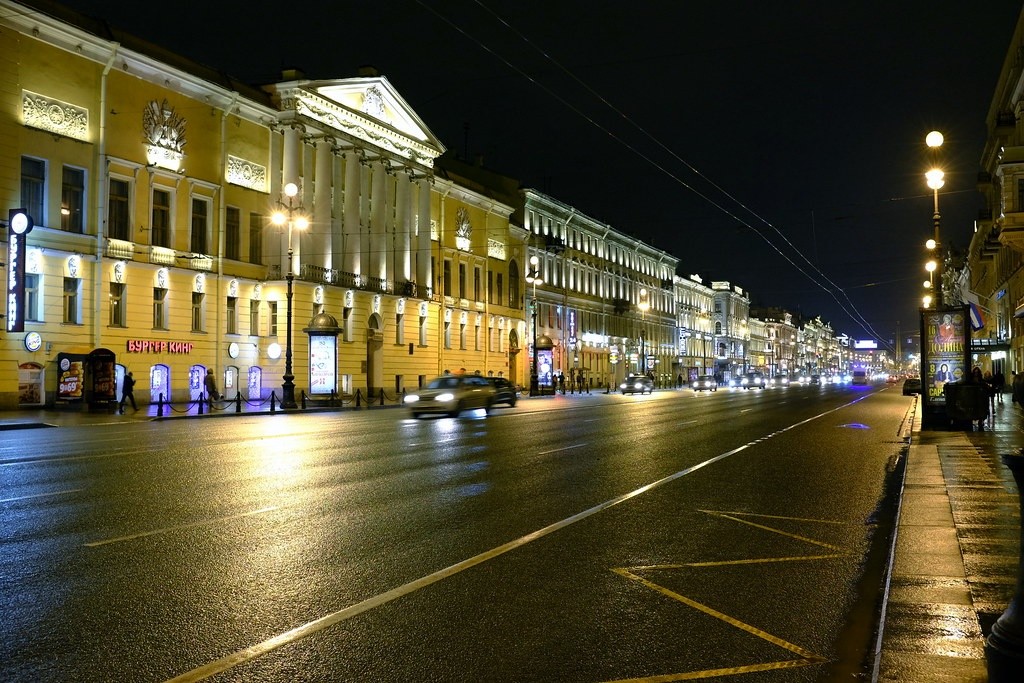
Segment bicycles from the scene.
[515,385,529,395]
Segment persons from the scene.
[970,367,989,432]
[933,364,957,388]
[678,375,682,388]
[953,367,966,383]
[995,369,1005,404]
[983,370,996,414]
[553,375,557,389]
[1012,371,1024,409]
[537,353,549,370]
[558,372,565,391]
[939,314,955,342]
[119,372,140,411]
[204,368,217,410]
[543,371,552,386]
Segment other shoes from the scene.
[134,408,140,411]
[119,407,123,414]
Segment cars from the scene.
[729,377,745,389]
[484,377,517,407]
[742,372,766,390]
[770,375,790,387]
[799,367,919,387]
[620,376,654,395]
[404,376,496,419]
[693,375,718,392]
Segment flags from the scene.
[969,302,987,329]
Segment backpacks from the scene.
[552,376,557,382]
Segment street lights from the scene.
[926,239,936,309]
[527,256,542,397]
[700,308,710,374]
[926,131,945,310]
[790,335,795,377]
[638,289,649,375]
[273,182,309,409]
[770,328,774,378]
[739,319,748,374]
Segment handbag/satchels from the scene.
[212,391,220,401]
